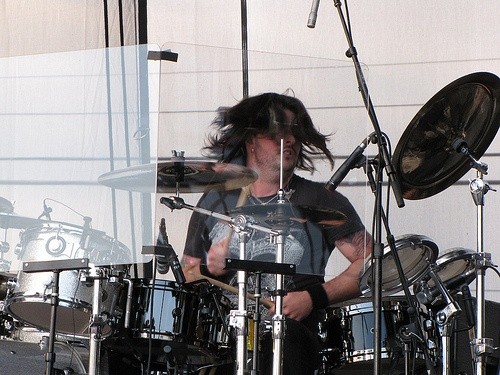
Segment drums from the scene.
[308,295,420,372]
[413,246,492,304]
[8,222,133,339]
[118,277,233,367]
[358,233,440,296]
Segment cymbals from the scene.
[97,158,260,193]
[391,71,500,201]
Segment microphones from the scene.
[44,200,51,221]
[307,0,320,29]
[156,218,170,274]
[325,136,370,193]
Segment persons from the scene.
[180,93,376,375]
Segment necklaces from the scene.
[246,185,288,206]
